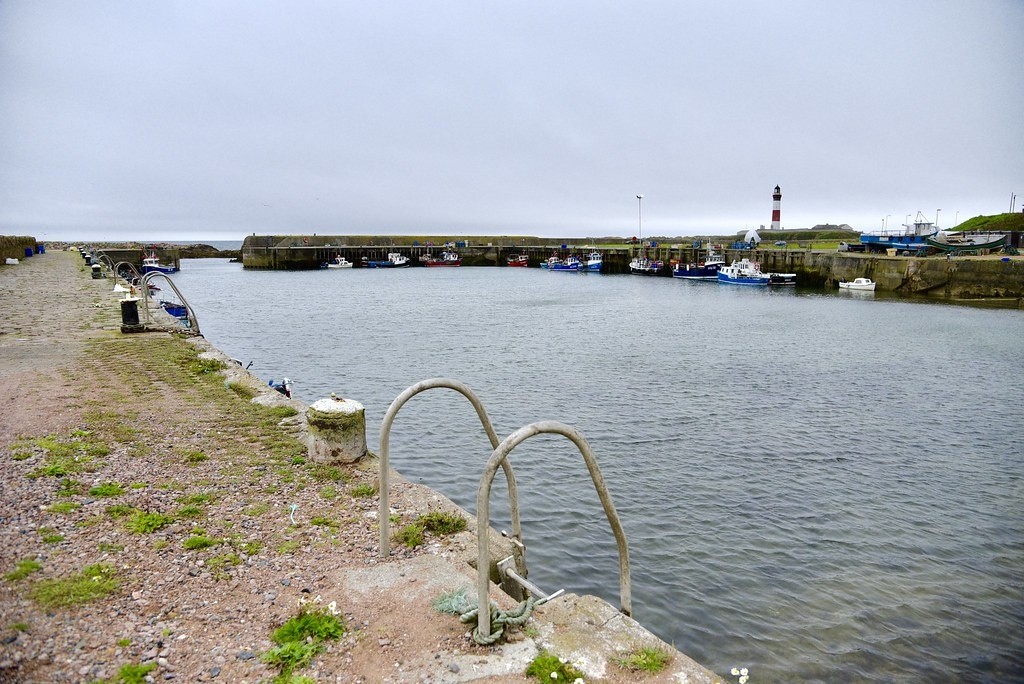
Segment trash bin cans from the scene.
[465,240,468,247]
[562,244,566,248]
[37,245,45,254]
[886,249,897,257]
[25,248,33,257]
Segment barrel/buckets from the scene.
[38,245,44,254]
[26,248,33,256]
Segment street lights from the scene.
[885,214,892,230]
[637,194,645,245]
[955,211,959,225]
[936,209,941,225]
[906,214,911,224]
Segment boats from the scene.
[858,219,942,253]
[143,251,178,275]
[120,270,189,327]
[506,253,529,266]
[672,238,726,280]
[927,230,1008,253]
[539,238,604,272]
[629,253,673,278]
[328,255,353,268]
[839,278,876,291]
[369,252,411,268]
[425,251,464,267]
[767,273,798,285]
[717,258,770,286]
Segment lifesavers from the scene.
[648,263,651,267]
[920,236,925,240]
[910,237,914,241]
[889,235,894,241]
[632,237,637,241]
[898,237,903,241]
[754,265,759,270]
[589,253,592,257]
[303,237,308,243]
